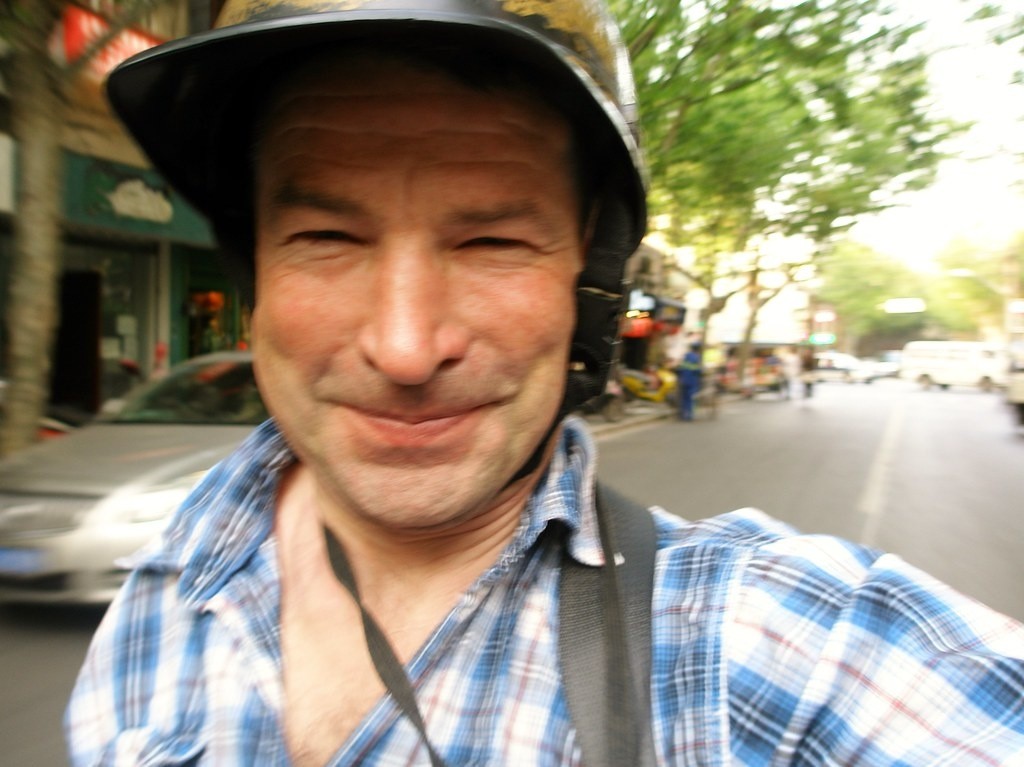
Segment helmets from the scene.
[101,0,649,417]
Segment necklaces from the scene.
[324,478,628,766]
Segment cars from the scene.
[0,346,268,611]
[815,352,899,385]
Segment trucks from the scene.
[901,340,1013,392]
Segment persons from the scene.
[676,342,704,420]
[66,0,1024,766]
